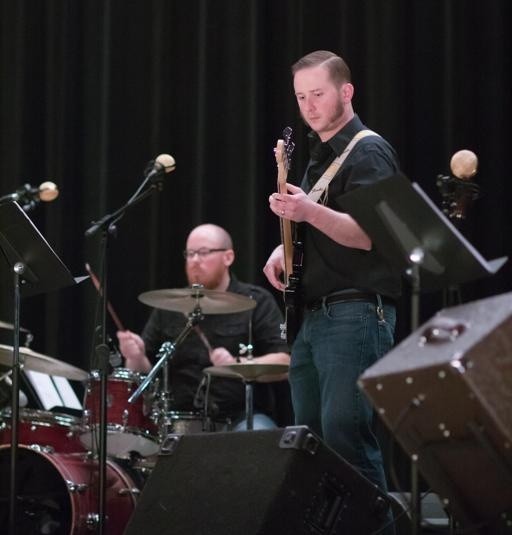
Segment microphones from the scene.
[146,154,177,180]
[450,149,479,218]
[20,181,59,211]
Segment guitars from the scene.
[273,126,306,343]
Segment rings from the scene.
[280,209,285,216]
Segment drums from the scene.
[0,443,137,535]
[134,412,230,473]
[0,404,83,452]
[79,369,164,459]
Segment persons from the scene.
[259,47,409,533]
[115,221,291,439]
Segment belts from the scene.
[304,291,397,312]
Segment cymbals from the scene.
[137,287,257,316]
[204,364,290,381]
[0,321,30,333]
[1,344,89,382]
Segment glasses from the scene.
[183,246,227,260]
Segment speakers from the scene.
[123,426,412,535]
[358,293,511,534]
